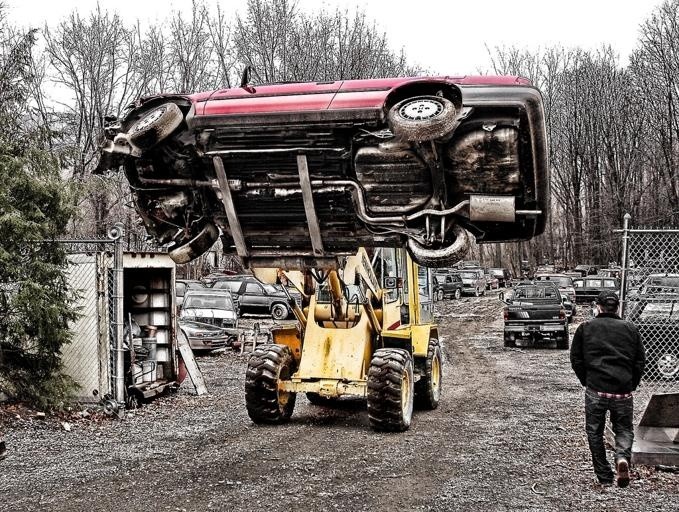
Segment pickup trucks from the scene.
[498,280,570,350]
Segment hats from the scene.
[594,289,620,306]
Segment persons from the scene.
[570,290,645,487]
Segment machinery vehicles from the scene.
[244,243,443,434]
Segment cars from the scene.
[103,66,553,267]
[625,292,679,378]
[176,263,513,321]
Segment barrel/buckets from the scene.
[133,364,143,385]
[144,324,158,338]
[132,337,142,362]
[142,337,158,361]
[142,363,158,383]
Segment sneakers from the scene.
[593,475,612,488]
[616,458,632,489]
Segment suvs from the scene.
[174,288,239,351]
[533,264,679,311]
